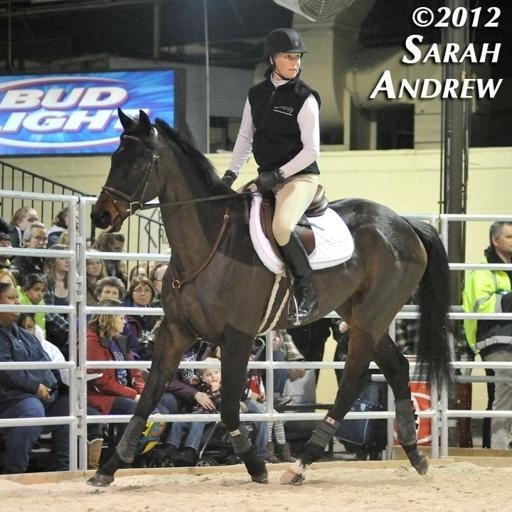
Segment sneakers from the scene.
[127,445,196,467]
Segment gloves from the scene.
[254,168,284,197]
[221,169,237,187]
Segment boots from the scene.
[279,231,320,323]
[86,438,104,469]
[265,442,297,462]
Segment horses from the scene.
[87,106,462,487]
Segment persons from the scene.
[219,25,324,319]
[462,221,512,450]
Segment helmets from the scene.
[263,27,311,58]
